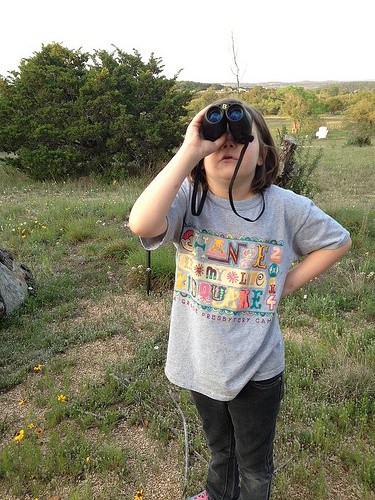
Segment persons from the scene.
[129,99,352,499]
